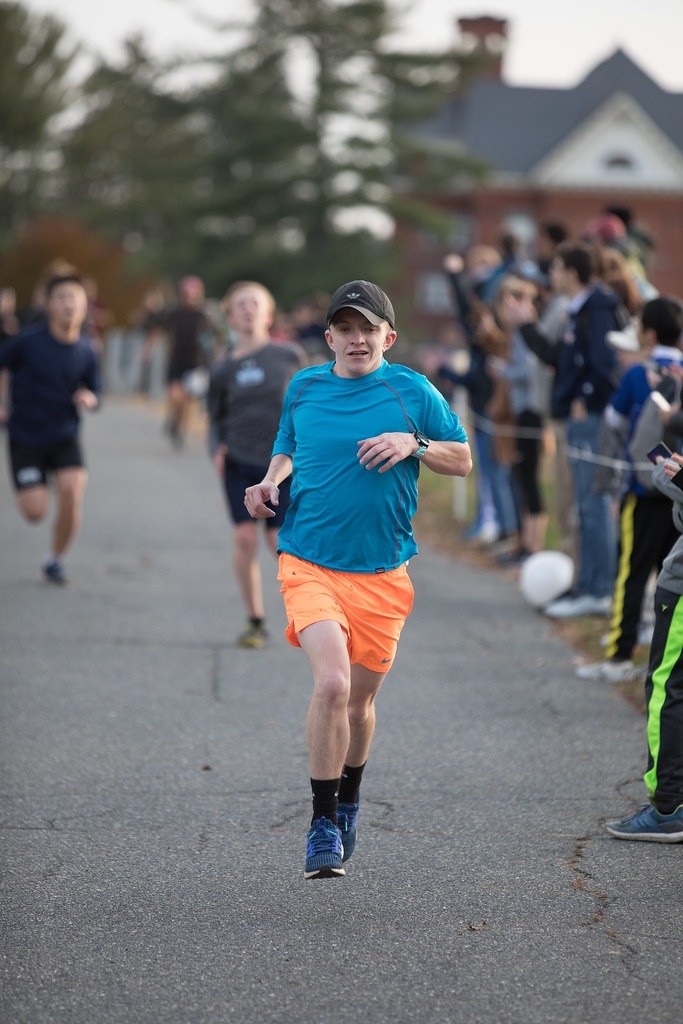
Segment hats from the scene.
[326,280,396,328]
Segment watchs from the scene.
[410,429,429,458]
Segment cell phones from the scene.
[646,441,673,466]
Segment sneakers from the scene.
[304,815,346,879]
[336,792,359,863]
[607,804,683,843]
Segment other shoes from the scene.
[237,617,269,650]
[546,595,613,619]
[577,660,634,684]
[42,564,64,582]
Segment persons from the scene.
[139,202,683,880]
[1,260,106,588]
